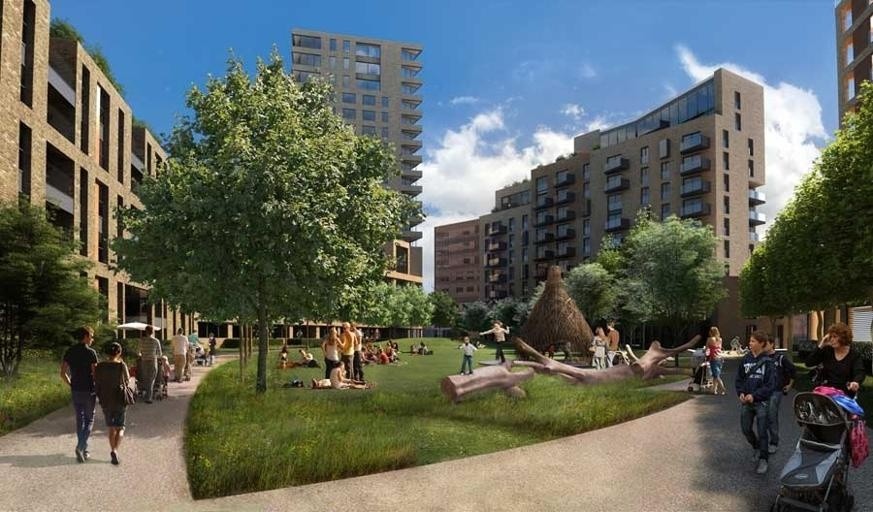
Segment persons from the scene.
[62,325,219,466]
[280,318,872,511]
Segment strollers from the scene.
[195,343,210,368]
[131,356,169,401]
[686,344,726,394]
[175,352,193,381]
[770,384,862,511]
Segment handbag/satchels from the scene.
[116,376,137,406]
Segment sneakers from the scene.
[75,447,90,463]
[768,443,777,454]
[751,448,761,462]
[111,449,120,466]
[756,458,769,475]
